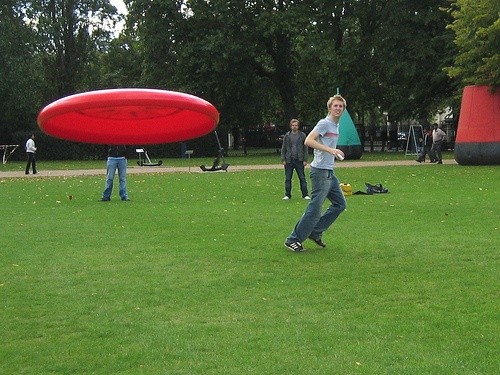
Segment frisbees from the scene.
[37,88,219,146]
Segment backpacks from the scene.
[366,183,388,193]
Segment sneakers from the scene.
[284,240,306,252]
[433,160,438,163]
[283,196,289,200]
[437,161,442,164]
[122,197,130,201]
[304,195,311,200]
[98,197,110,201]
[309,235,326,248]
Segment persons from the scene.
[98,143,130,202]
[24,134,39,174]
[284,95,347,252]
[416,126,433,162]
[281,119,311,199]
[430,123,446,164]
